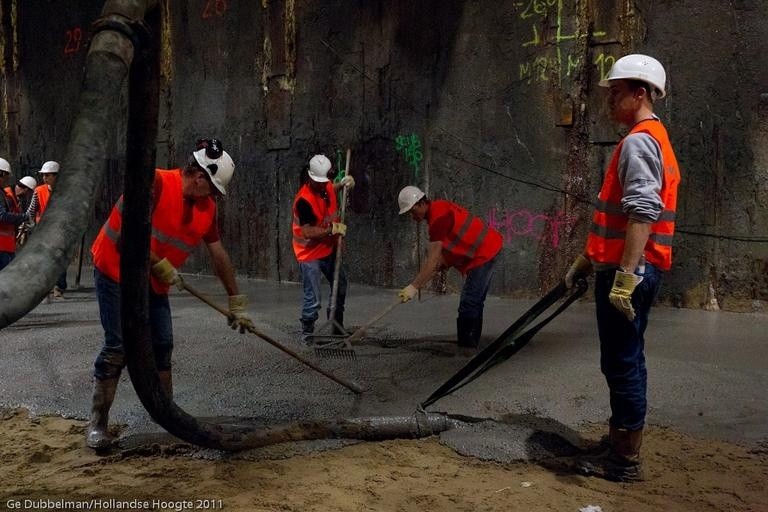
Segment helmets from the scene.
[597,49,671,97]
[37,158,59,175]
[19,173,39,192]
[305,152,332,183]
[0,158,14,175]
[192,144,240,200]
[391,184,426,215]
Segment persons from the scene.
[0,157,28,268]
[395,184,504,348]
[565,52,682,480]
[291,154,355,337]
[87,144,253,448]
[10,176,36,212]
[21,161,67,297]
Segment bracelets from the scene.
[617,265,627,272]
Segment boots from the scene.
[456,316,484,347]
[326,304,347,336]
[602,423,647,483]
[52,285,66,303]
[86,374,119,448]
[301,315,317,348]
[155,371,200,425]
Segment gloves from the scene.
[564,252,595,289]
[609,269,645,322]
[396,282,421,303]
[226,293,256,334]
[148,256,185,292]
[331,220,348,237]
